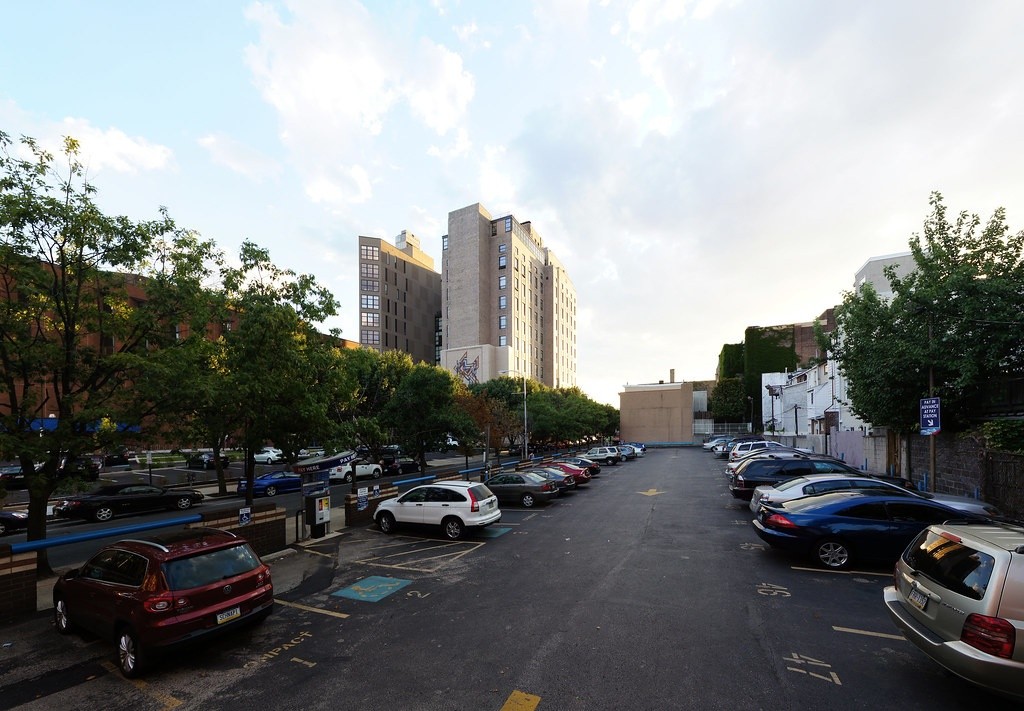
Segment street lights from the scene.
[766,384,781,436]
[748,397,754,423]
[498,369,531,462]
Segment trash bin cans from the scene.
[85,464,99,482]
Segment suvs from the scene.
[577,446,622,466]
[882,519,1022,695]
[376,481,505,540]
[187,450,230,471]
[729,453,920,502]
[48,526,273,681]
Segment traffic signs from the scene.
[921,397,945,437]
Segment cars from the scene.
[624,442,649,452]
[536,461,591,486]
[617,447,635,462]
[51,482,205,521]
[100,446,130,466]
[480,472,559,507]
[0,512,28,534]
[0,466,45,489]
[33,456,100,481]
[251,448,289,465]
[523,465,577,496]
[622,444,644,457]
[749,487,994,571]
[749,474,1001,520]
[331,457,384,484]
[702,433,846,485]
[293,447,328,459]
[556,457,602,477]
[236,470,302,498]
[374,455,423,475]
